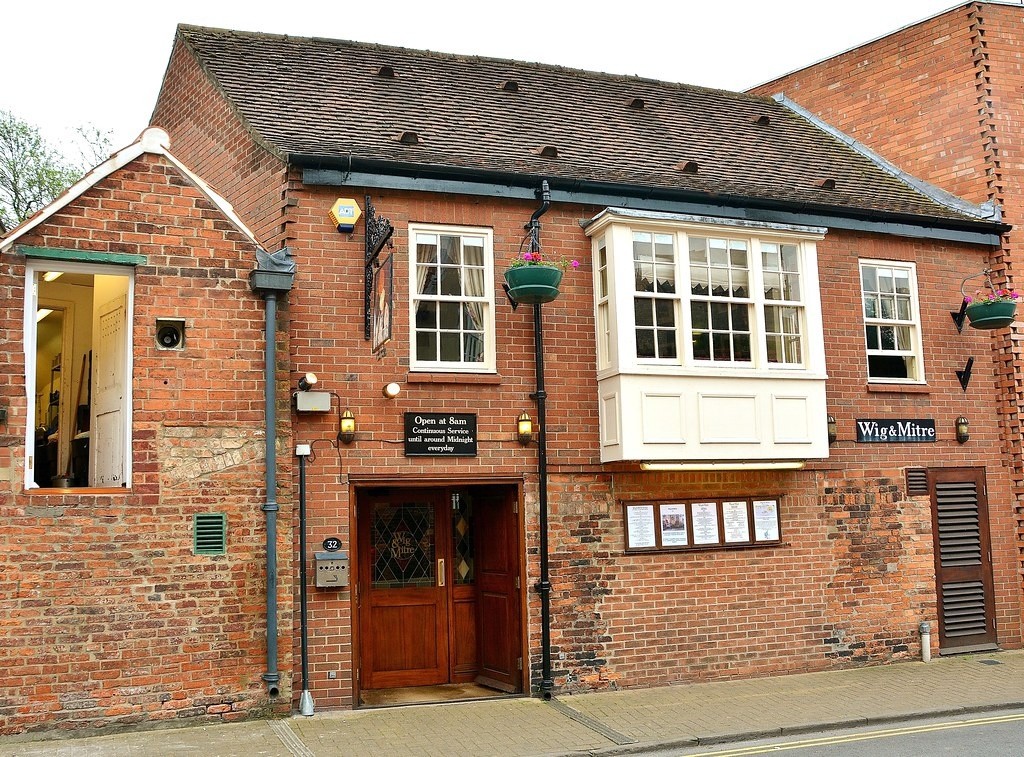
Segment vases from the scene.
[964,299,1017,330]
[503,265,563,304]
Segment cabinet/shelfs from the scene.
[47,363,63,436]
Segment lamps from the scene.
[516,409,533,447]
[827,413,837,446]
[337,408,356,446]
[298,373,318,392]
[955,414,969,445]
[382,381,400,400]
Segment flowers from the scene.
[509,253,580,271]
[963,288,1019,306]
